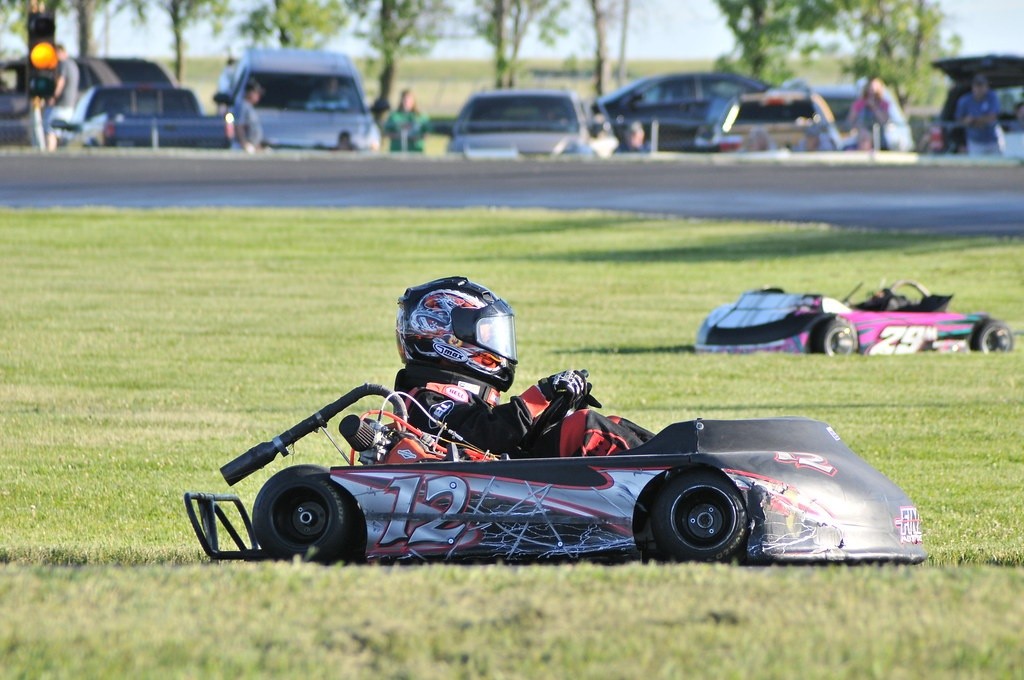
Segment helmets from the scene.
[394,274,519,393]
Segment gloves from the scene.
[540,369,587,399]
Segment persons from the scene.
[26,44,80,147]
[395,276,655,458]
[790,125,832,150]
[846,78,889,149]
[338,132,355,150]
[845,129,875,149]
[615,122,650,152]
[214,59,235,113]
[231,79,262,150]
[956,76,1001,155]
[736,125,775,153]
[1009,103,1024,130]
[384,91,431,151]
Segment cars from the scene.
[422,90,612,162]
[719,91,839,151]
[597,72,776,156]
[921,55,1024,160]
[1,56,175,147]
[40,85,227,151]
[214,47,382,158]
[783,83,908,153]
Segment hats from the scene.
[973,72,985,85]
